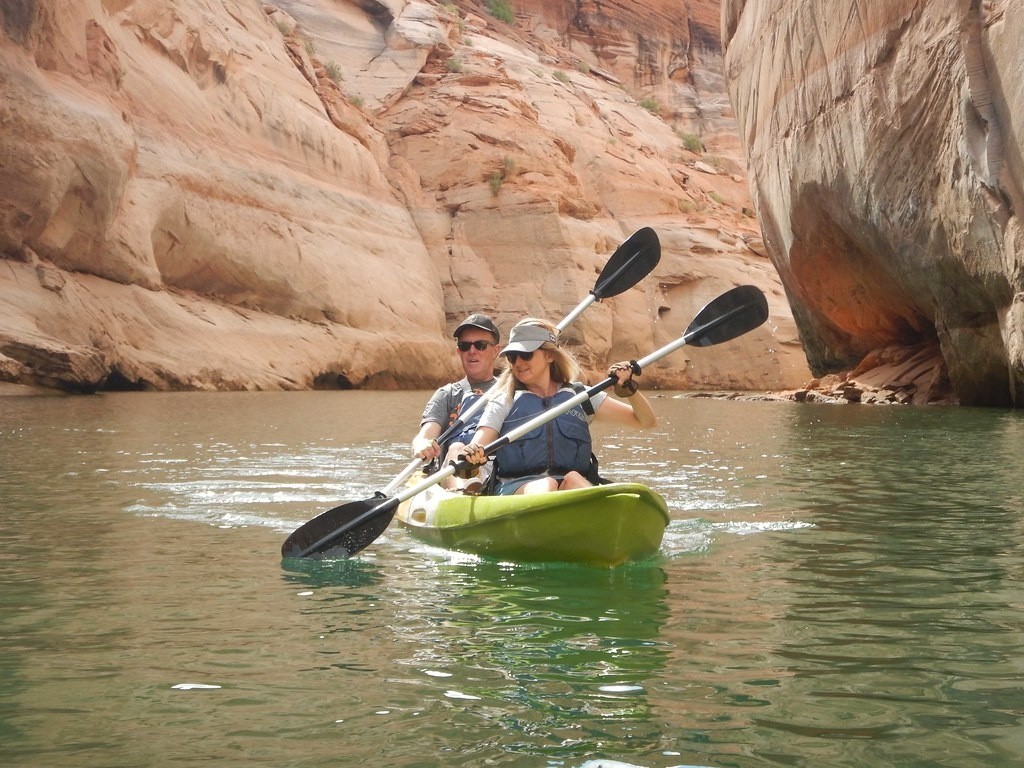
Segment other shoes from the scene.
[463,477,482,496]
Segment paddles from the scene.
[281,284,770,562]
[368,224,663,501]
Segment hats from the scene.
[453,315,500,345]
[498,325,556,358]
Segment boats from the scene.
[392,458,671,571]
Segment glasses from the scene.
[457,340,495,353]
[505,349,547,363]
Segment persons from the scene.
[458,318,657,495]
[412,314,502,496]
